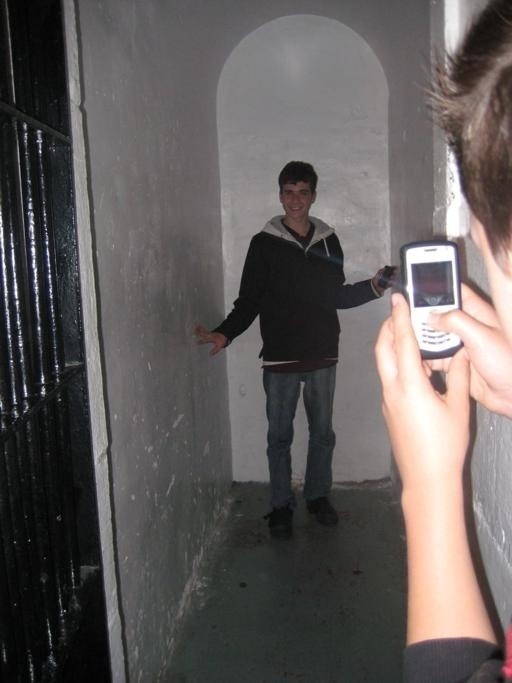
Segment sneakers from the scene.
[307,497,338,526]
[271,508,292,539]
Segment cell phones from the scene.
[378,265,394,288]
[400,241,464,359]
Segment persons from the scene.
[374,0,512,683]
[192,160,398,542]
